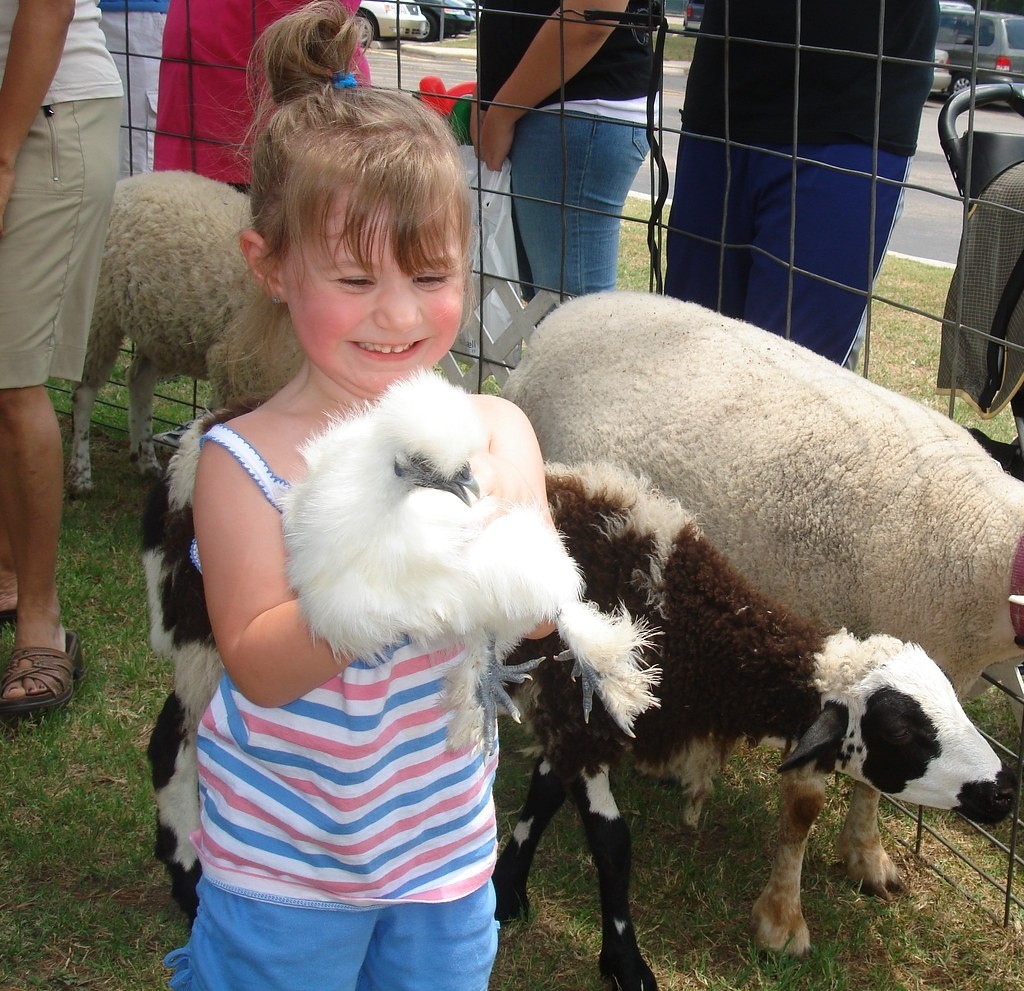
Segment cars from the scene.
[927,48,953,95]
[682,0,705,37]
[934,9,1024,101]
[938,1,975,12]
[353,0,483,47]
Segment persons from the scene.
[168,8,559,991]
[99,0,169,179]
[662,0,940,372]
[470,0,668,304]
[153,0,369,184]
[0,0,123,708]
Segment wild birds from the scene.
[277,368,664,768]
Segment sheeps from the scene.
[138,401,1019,991]
[66,169,305,503]
[502,288,1024,958]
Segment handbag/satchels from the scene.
[451,143,523,367]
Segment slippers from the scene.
[0,631,84,714]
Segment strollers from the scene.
[936,79,1024,483]
